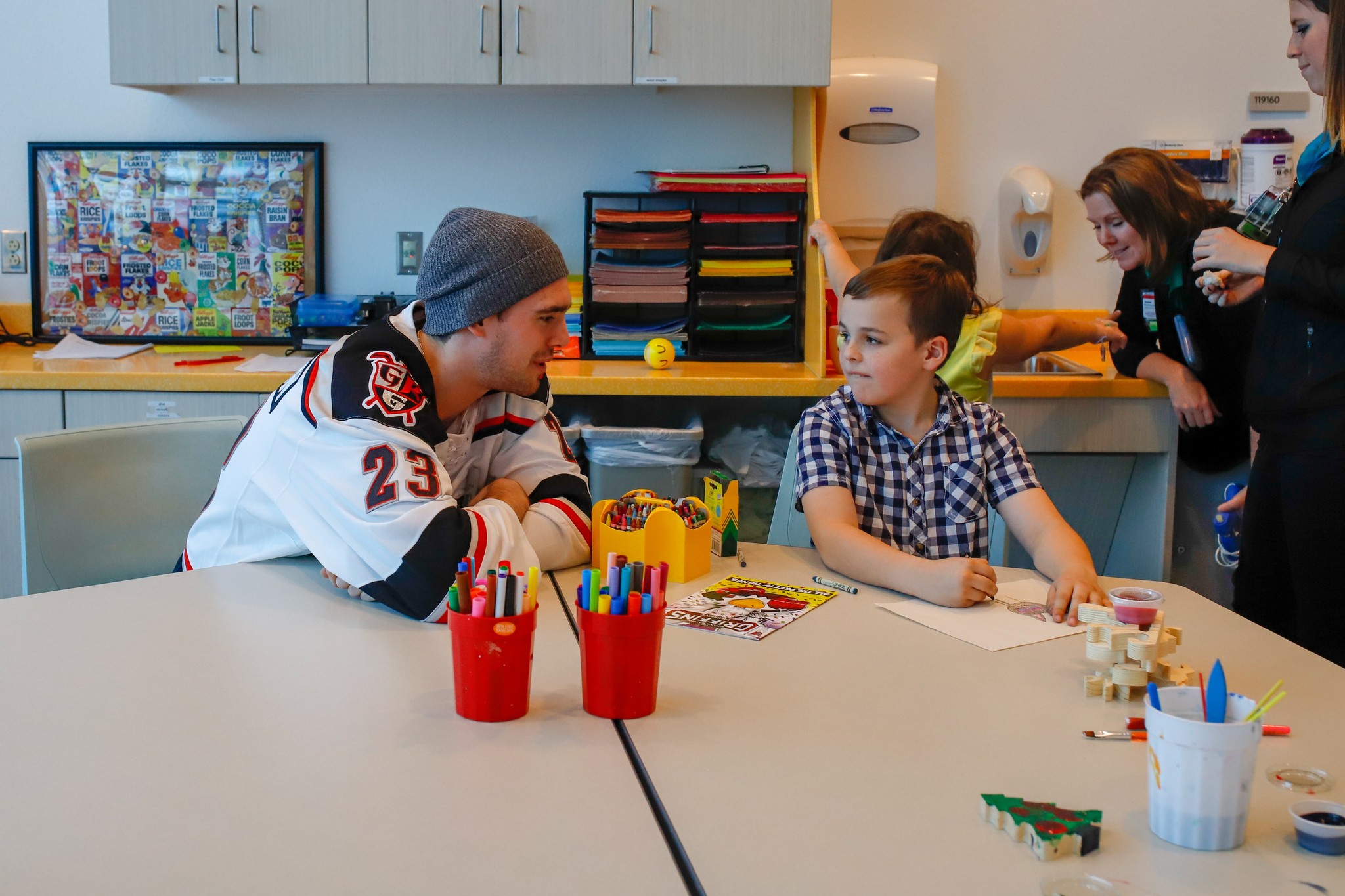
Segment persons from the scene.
[173,206,591,624]
[1081,149,1274,511]
[808,212,1126,405]
[796,254,1112,626]
[1191,0,1344,665]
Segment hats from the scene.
[414,206,571,338]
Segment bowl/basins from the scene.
[1108,587,1166,625]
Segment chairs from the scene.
[767,419,1011,568]
[13,412,250,598]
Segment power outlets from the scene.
[1,230,27,274]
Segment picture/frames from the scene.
[27,142,325,346]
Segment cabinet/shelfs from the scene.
[366,0,633,85]
[0,390,276,601]
[580,190,809,362]
[107,0,367,85]
[632,0,832,86]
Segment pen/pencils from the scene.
[1126,717,1290,736]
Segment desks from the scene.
[551,539,1345,896]
[0,553,692,896]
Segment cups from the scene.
[1143,686,1263,851]
[573,597,668,720]
[446,599,539,723]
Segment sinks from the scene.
[987,347,1108,380]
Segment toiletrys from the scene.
[996,165,1056,279]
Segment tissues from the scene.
[812,55,943,258]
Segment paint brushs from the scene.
[1083,731,1147,743]
